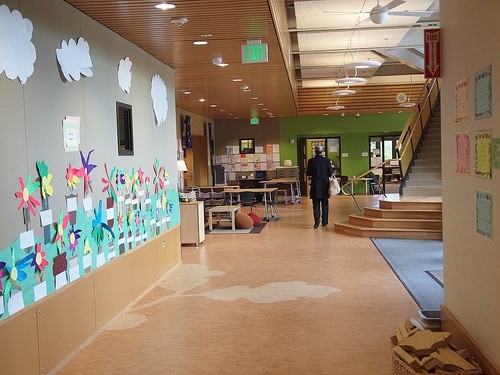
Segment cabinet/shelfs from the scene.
[276,165,299,196]
[186,185,241,223]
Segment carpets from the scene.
[204,222,267,234]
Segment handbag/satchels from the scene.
[328,173,340,196]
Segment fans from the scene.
[322,0,434,26]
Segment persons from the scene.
[306,146,332,229]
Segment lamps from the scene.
[332,63,356,96]
[177,159,188,192]
[398,74,416,108]
[341,20,381,70]
[325,85,346,110]
[334,43,366,84]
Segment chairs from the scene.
[368,174,381,195]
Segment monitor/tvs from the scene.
[256,171,266,178]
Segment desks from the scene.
[222,189,278,222]
[348,178,373,196]
[259,179,301,205]
[209,205,241,232]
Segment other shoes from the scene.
[314,219,320,228]
